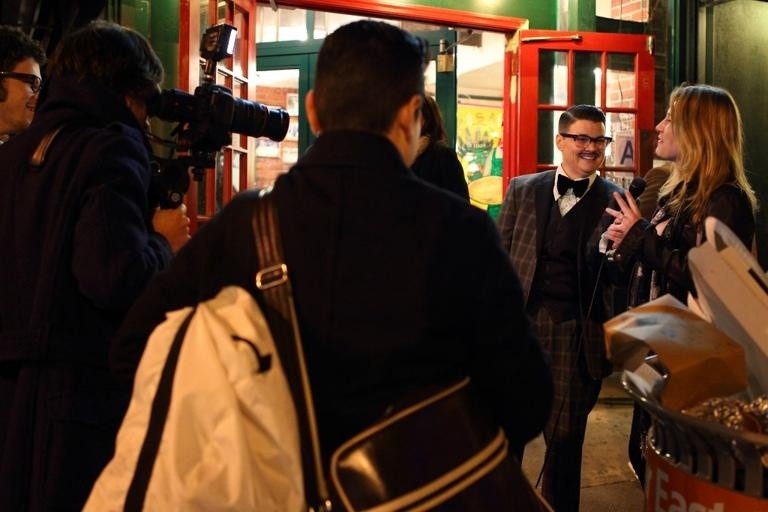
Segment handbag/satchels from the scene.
[308,375,556,512]
[80,188,306,512]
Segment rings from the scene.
[620,215,626,220]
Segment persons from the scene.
[1,19,196,511]
[93,18,555,512]
[497,105,633,512]
[408,95,471,206]
[595,85,759,486]
[0,23,47,143]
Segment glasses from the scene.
[560,132,612,150]
[0,72,41,93]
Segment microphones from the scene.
[606,176,647,253]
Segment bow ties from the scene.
[557,174,589,198]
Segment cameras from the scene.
[154,23,290,172]
